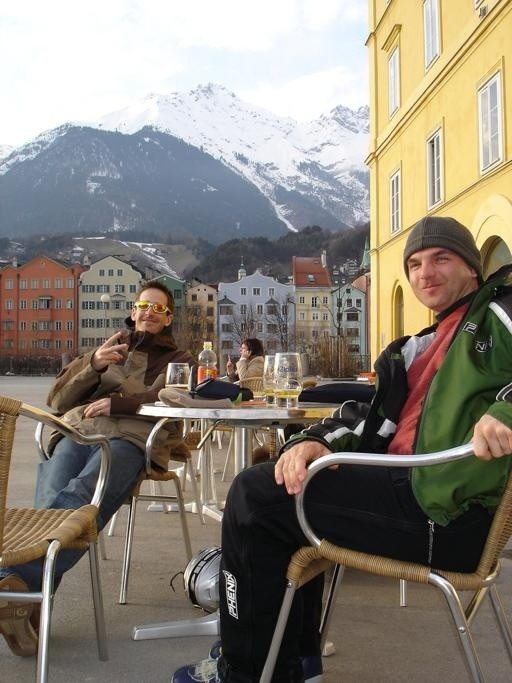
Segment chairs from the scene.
[261,443,510,683]
[1,396,114,683]
[35,410,195,607]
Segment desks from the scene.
[134,395,356,647]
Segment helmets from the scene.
[182,545,223,615]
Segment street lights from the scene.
[337,334,343,377]
[328,334,335,376]
[100,293,112,340]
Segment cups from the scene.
[262,351,303,410]
[165,361,191,392]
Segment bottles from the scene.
[197,340,218,386]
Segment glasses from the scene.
[132,300,173,316]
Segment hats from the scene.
[403,215,485,289]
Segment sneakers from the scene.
[0,573,42,658]
[169,640,325,683]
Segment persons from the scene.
[227,337,265,382]
[0,278,196,658]
[173,216,512,683]
